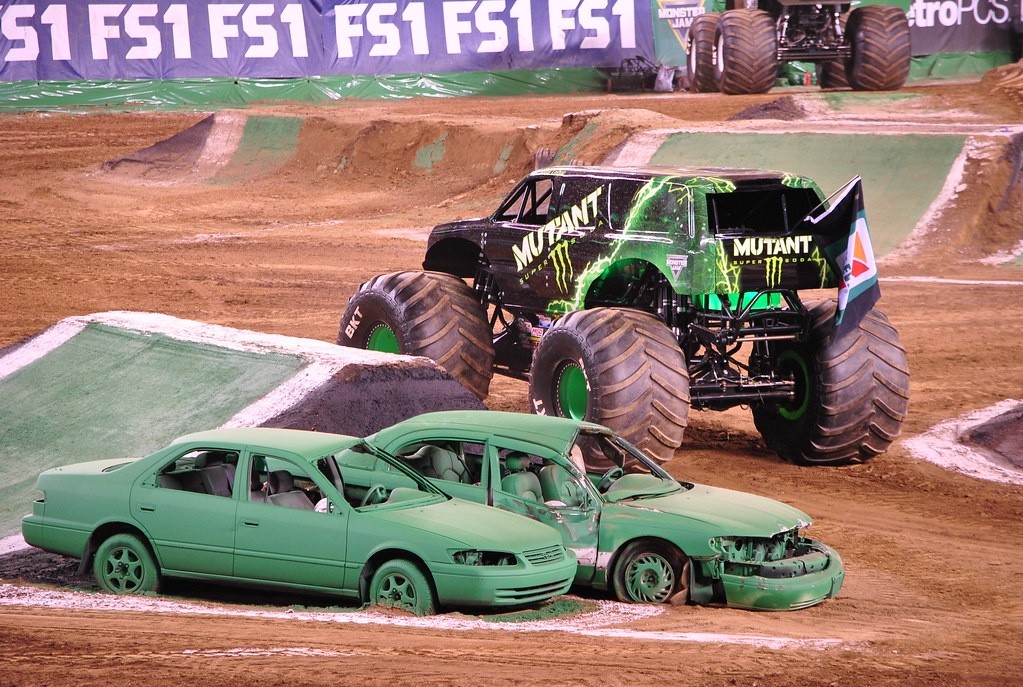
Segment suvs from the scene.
[335,162,910,470]
[686,0,911,94]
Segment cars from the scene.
[21,426,578,614]
[359,410,846,614]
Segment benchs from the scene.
[159,453,267,504]
[399,445,472,486]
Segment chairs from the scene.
[539,458,583,506]
[501,452,545,504]
[266,470,315,510]
